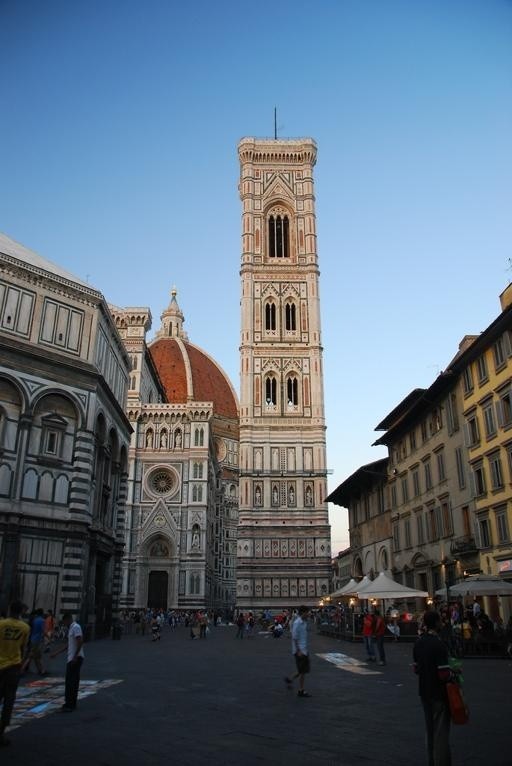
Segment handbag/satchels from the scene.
[447,682,470,725]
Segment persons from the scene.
[1,601,57,746]
[412,610,455,766]
[285,607,313,697]
[371,609,388,665]
[119,606,337,642]
[61,613,86,715]
[361,606,375,666]
[331,599,511,654]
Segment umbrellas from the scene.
[434,573,512,597]
[330,572,429,618]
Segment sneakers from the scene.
[362,657,387,666]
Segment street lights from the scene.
[442,559,458,633]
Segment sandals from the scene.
[284,677,293,689]
[296,689,311,697]
